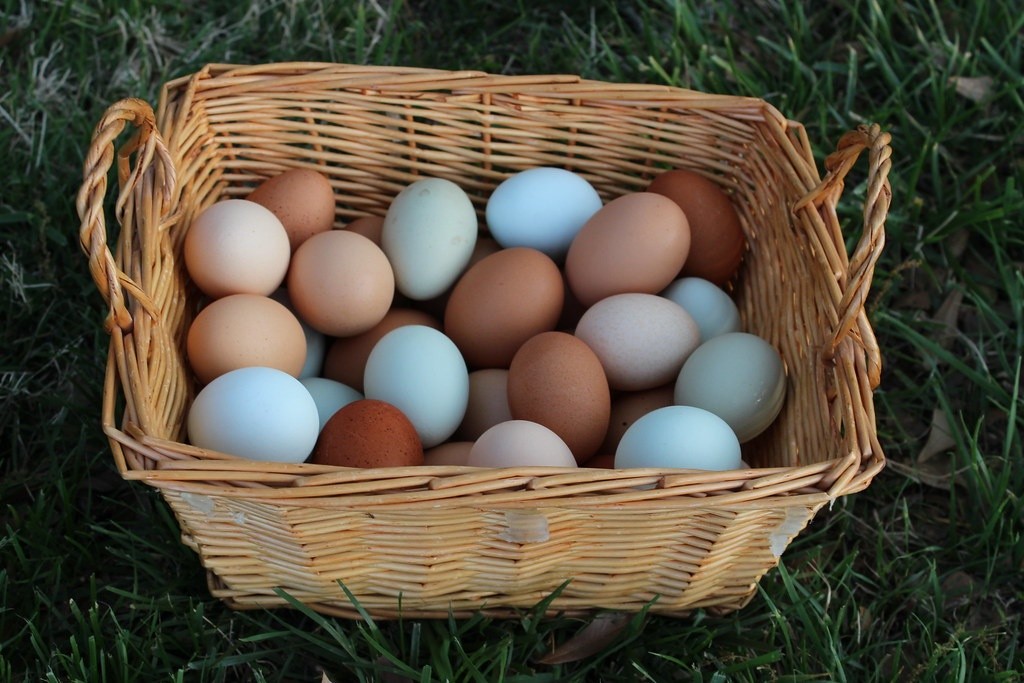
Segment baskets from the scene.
[77,63,892,620]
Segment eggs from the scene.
[183,167,787,471]
